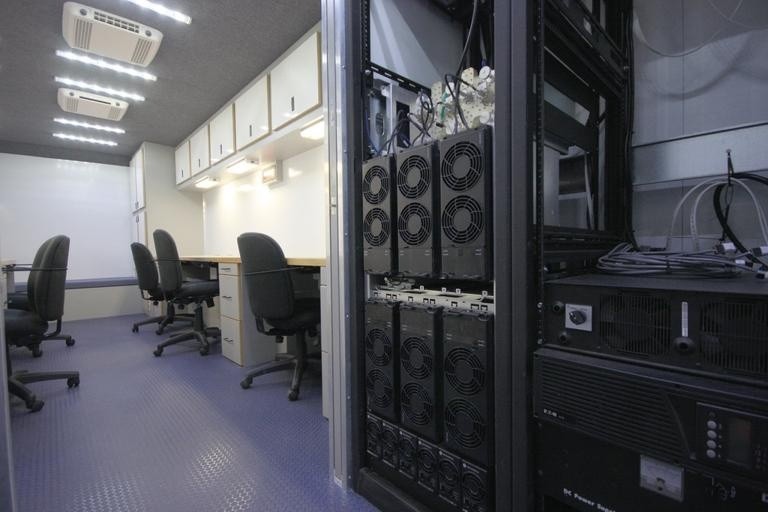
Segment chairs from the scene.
[0,235,81,412]
[128,227,320,402]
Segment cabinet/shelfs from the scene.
[172,19,327,193]
[128,141,206,256]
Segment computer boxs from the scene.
[437,126,491,283]
[543,272,768,387]
[361,298,402,426]
[360,152,396,280]
[396,303,441,445]
[439,307,495,475]
[395,142,438,283]
